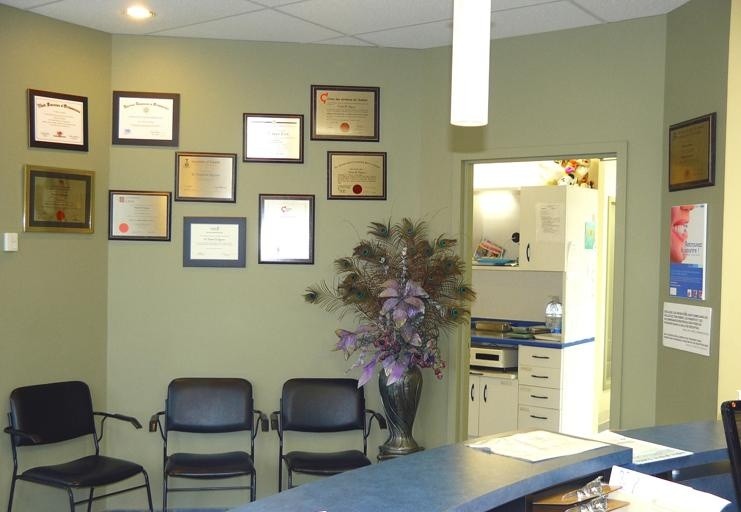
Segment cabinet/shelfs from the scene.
[519,185,565,271]
[467,375,516,438]
[516,345,559,432]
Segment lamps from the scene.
[450,0,492,128]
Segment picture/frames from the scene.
[107,190,170,241]
[258,194,315,264]
[112,91,179,148]
[23,163,95,234]
[665,112,716,192]
[243,112,304,164]
[183,216,247,268]
[174,152,237,204]
[28,88,89,153]
[327,151,387,199]
[309,84,379,142]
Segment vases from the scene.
[376,364,423,463]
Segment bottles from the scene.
[546,297,561,331]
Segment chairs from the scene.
[4,380,154,512]
[149,378,269,512]
[270,377,386,492]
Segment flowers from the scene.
[302,214,478,390]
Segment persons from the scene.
[671,206,698,263]
